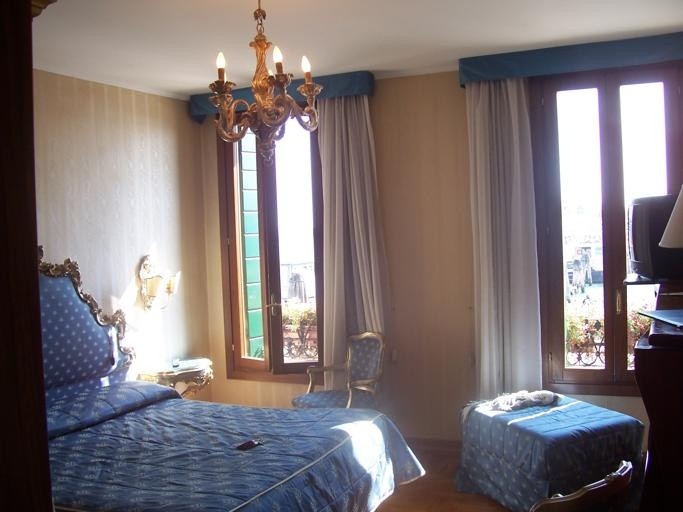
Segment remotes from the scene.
[238,439,261,450]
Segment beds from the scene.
[38,244,414,512]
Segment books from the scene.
[638,309,683,327]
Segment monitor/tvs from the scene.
[627,194,683,281]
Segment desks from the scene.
[136,357,214,400]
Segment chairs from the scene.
[291,332,386,408]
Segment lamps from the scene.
[657,181,683,249]
[136,254,181,312]
[209,0,325,163]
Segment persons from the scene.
[581,249,592,286]
[573,250,584,293]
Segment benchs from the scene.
[453,391,646,510]
